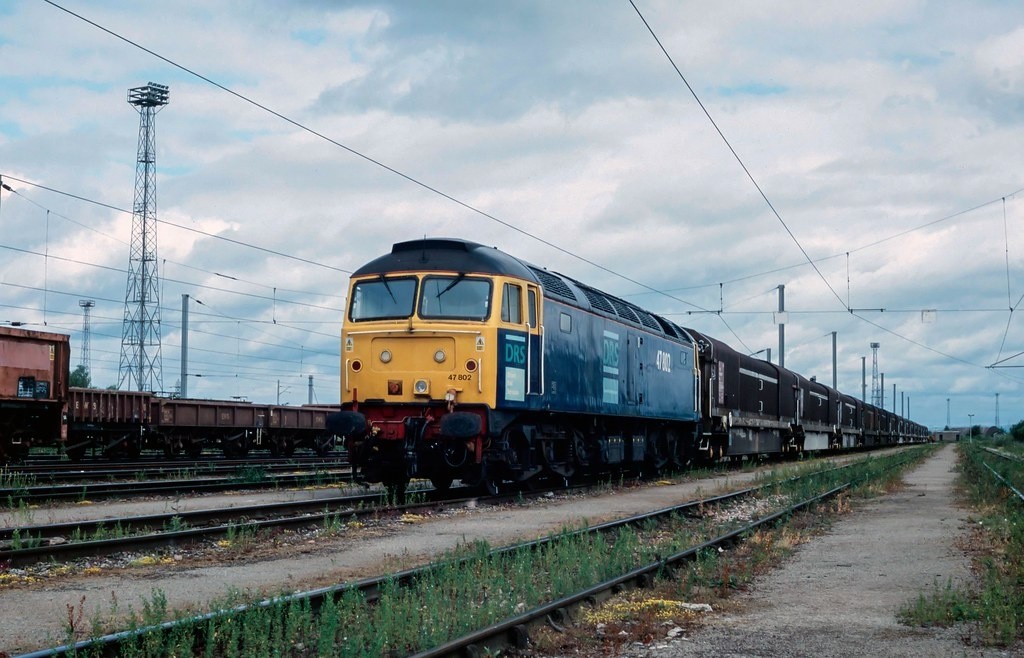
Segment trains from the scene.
[327,236,933,498]
[0,325,344,464]
